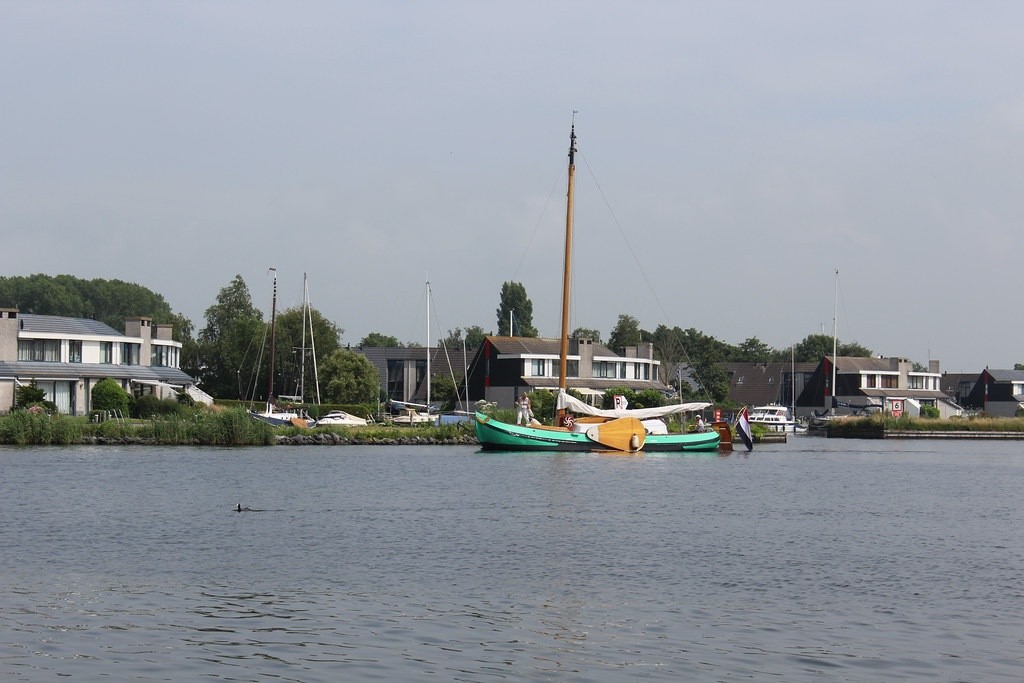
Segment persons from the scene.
[689,414,704,433]
[515,391,531,426]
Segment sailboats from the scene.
[810,264,882,427]
[473,110,721,452]
[237,267,322,429]
[383,271,475,423]
[749,340,807,433]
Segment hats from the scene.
[696,415,701,418]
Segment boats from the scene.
[317,409,369,428]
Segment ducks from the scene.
[236,503,251,512]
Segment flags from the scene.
[736,409,753,451]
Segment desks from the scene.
[689,424,712,434]
